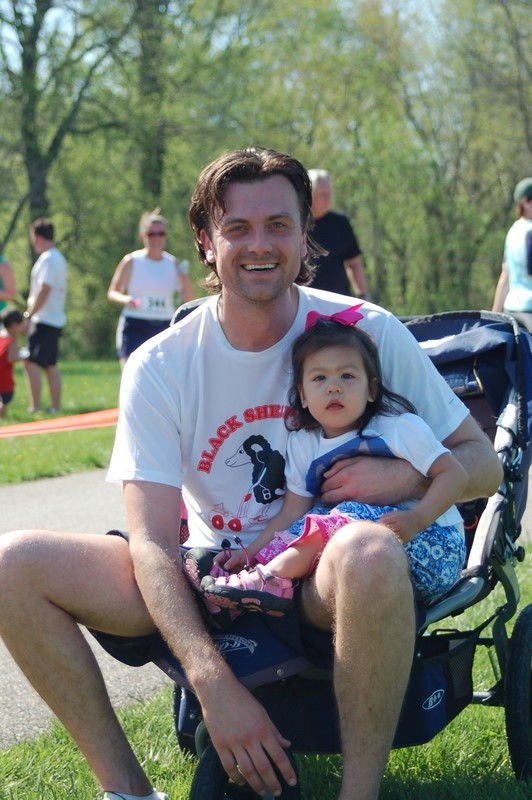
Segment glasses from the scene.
[148,230,166,237]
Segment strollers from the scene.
[173,310,532,800]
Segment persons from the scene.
[305,169,371,304]
[107,208,196,374]
[183,317,467,628]
[0,218,68,415]
[0,147,504,800]
[492,177,532,333]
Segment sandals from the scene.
[183,547,242,628]
[200,536,295,619]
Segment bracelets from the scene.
[23,312,31,318]
